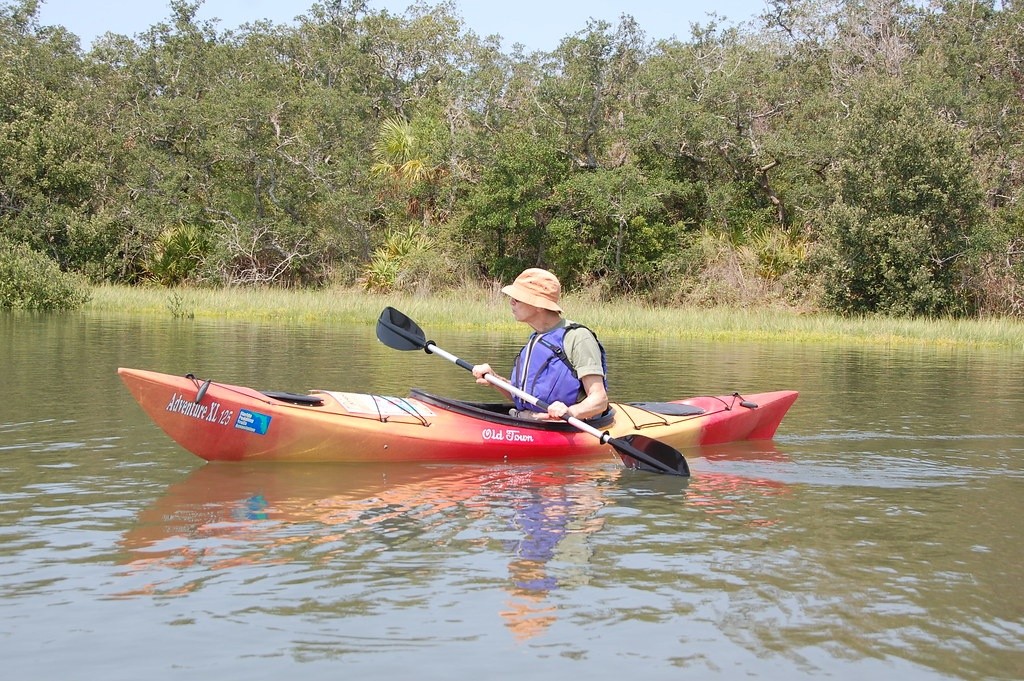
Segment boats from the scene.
[118,365,801,464]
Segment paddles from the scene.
[375,306,691,476]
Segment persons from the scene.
[473,267,609,424]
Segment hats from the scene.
[501,268,564,315]
